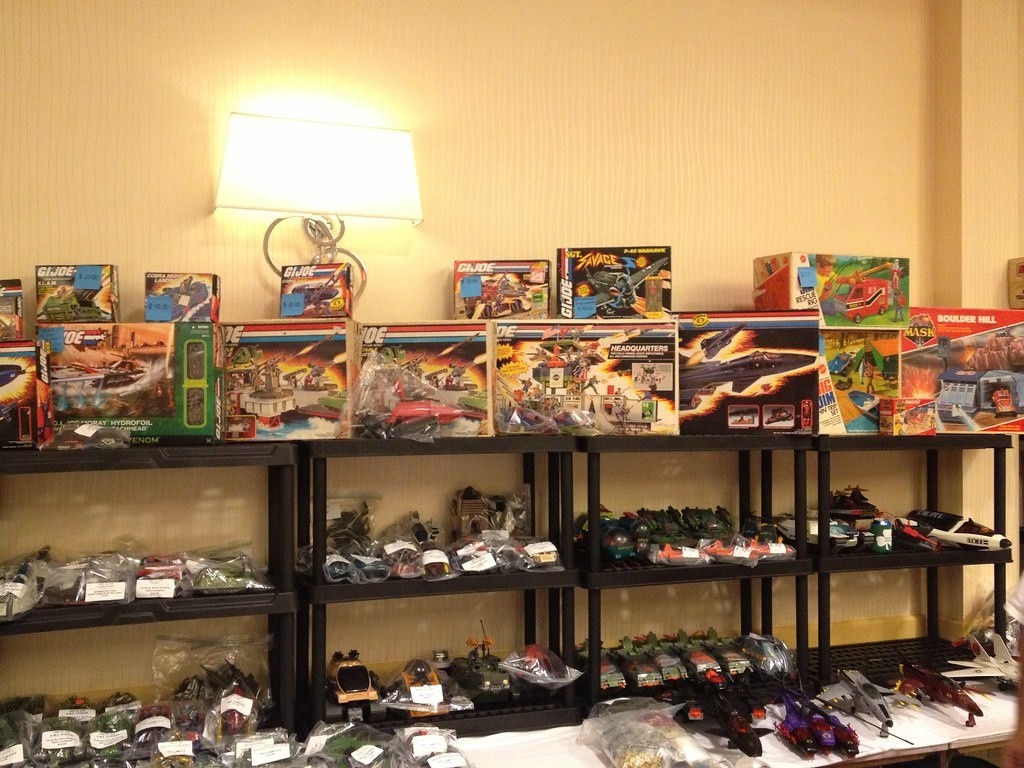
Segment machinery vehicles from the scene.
[326,615,799,727]
[0,485,560,623]
[573,485,1011,567]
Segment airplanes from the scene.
[656,634,1024,760]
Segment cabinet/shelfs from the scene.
[760,434,1014,700]
[561,438,818,721]
[299,436,580,746]
[0,441,298,768]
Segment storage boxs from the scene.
[362,320,498,437]
[279,262,354,318]
[878,398,937,438]
[144,273,222,324]
[35,323,223,450]
[0,340,36,451]
[0,279,23,342]
[901,307,1024,433]
[221,319,363,443]
[557,246,672,319]
[454,260,551,321]
[497,319,681,437]
[672,310,819,437]
[818,330,903,436]
[35,264,120,324]
[1008,257,1024,309]
[753,252,910,328]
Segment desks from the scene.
[748,676,1020,768]
